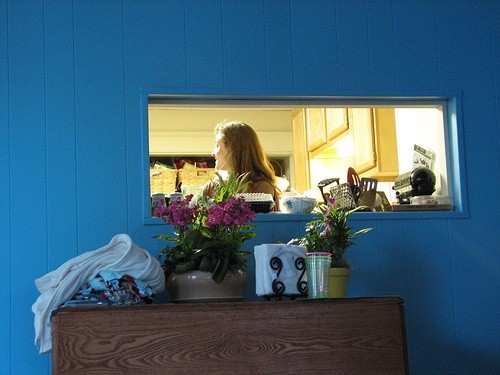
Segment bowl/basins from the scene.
[286,196,316,213]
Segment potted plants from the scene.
[286,198,374,298]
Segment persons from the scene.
[189,120,281,212]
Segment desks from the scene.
[50,296,410,375]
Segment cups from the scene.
[305,252,331,298]
[169,192,183,203]
[152,193,166,209]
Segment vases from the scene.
[165,269,247,302]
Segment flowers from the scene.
[148,172,256,284]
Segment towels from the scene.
[30,232,165,354]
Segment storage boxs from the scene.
[150,161,177,195]
[178,159,216,194]
[254,243,308,301]
